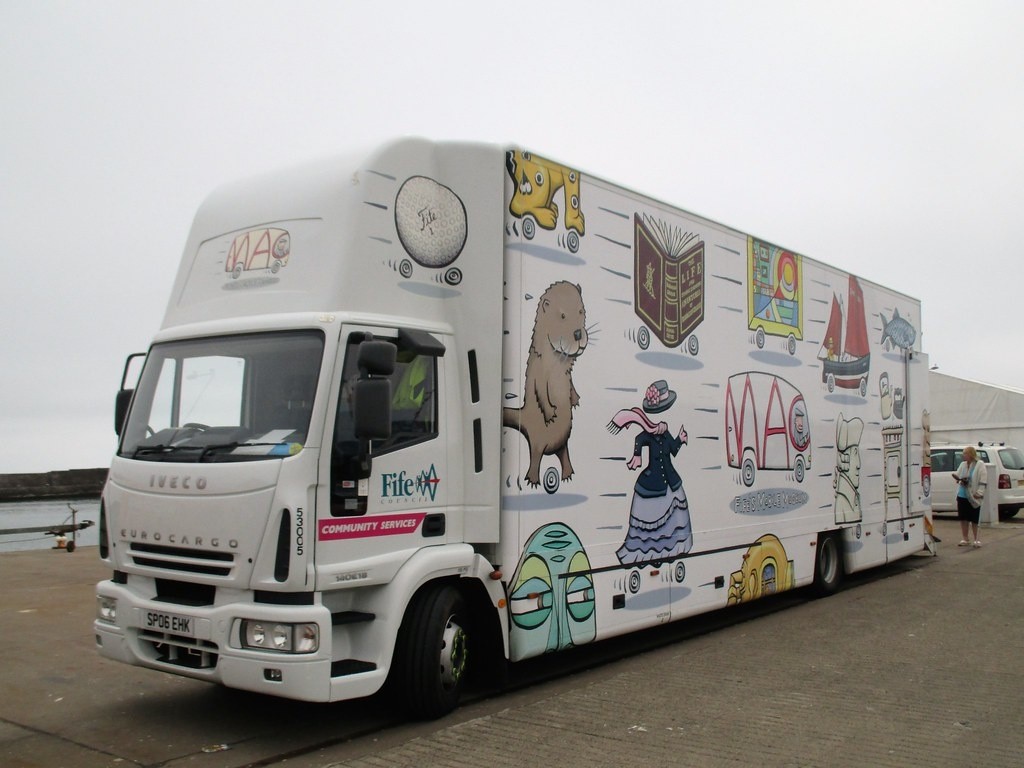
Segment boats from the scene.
[0,520,90,534]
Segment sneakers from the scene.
[973,541,982,548]
[958,541,969,546]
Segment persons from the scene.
[954,447,987,548]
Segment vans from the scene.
[929,442,1024,524]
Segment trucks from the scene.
[90,136,941,719]
